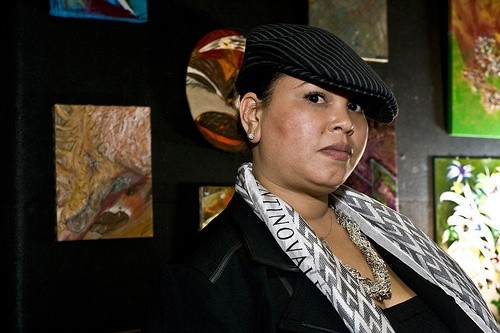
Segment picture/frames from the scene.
[430,155,500,328]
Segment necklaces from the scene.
[315,209,331,242]
[308,204,391,302]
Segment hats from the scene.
[235,23,399,125]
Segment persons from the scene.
[180,22,500,332]
